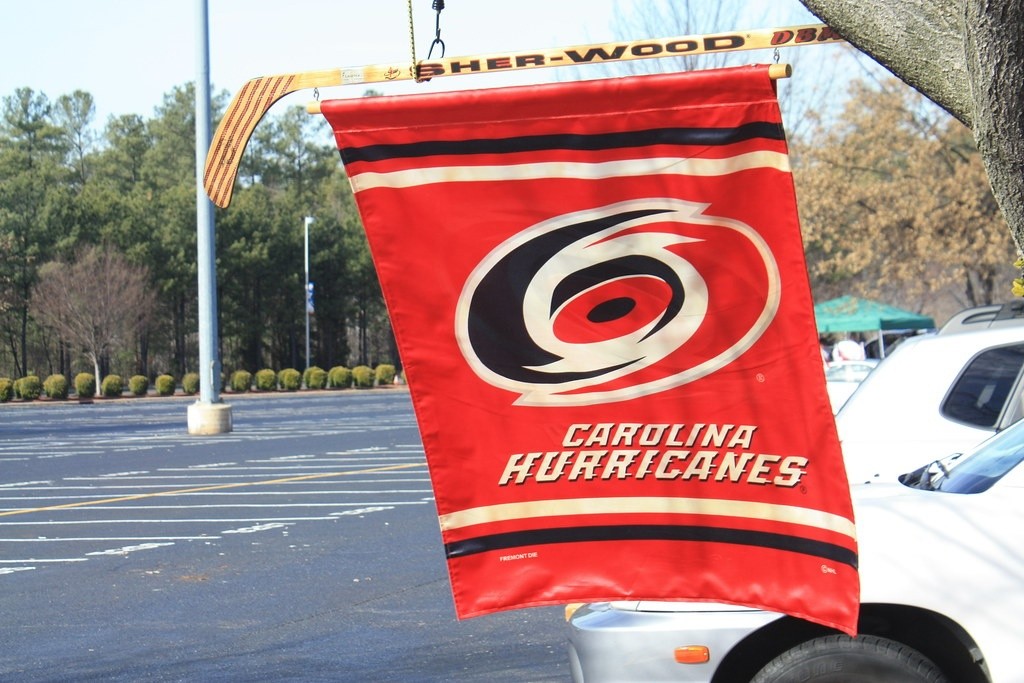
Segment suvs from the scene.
[835,300,1024,474]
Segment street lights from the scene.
[304,216,315,368]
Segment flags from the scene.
[320,64,861,637]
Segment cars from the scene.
[565,421,1024,683]
[824,361,881,413]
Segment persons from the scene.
[819,332,895,363]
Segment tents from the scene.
[813,295,935,359]
